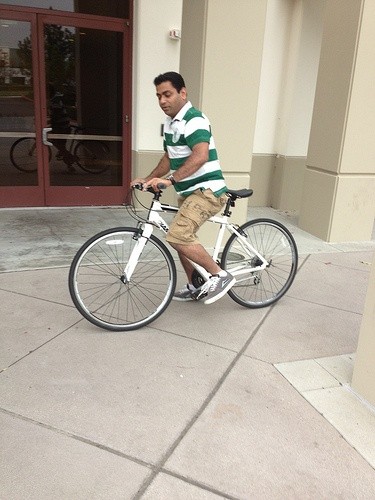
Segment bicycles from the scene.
[9,117,113,175]
[68,183,299,331]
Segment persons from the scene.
[45,83,71,156]
[129,71,238,304]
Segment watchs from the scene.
[166,174,176,185]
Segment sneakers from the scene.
[202,270,236,305]
[171,285,208,301]
